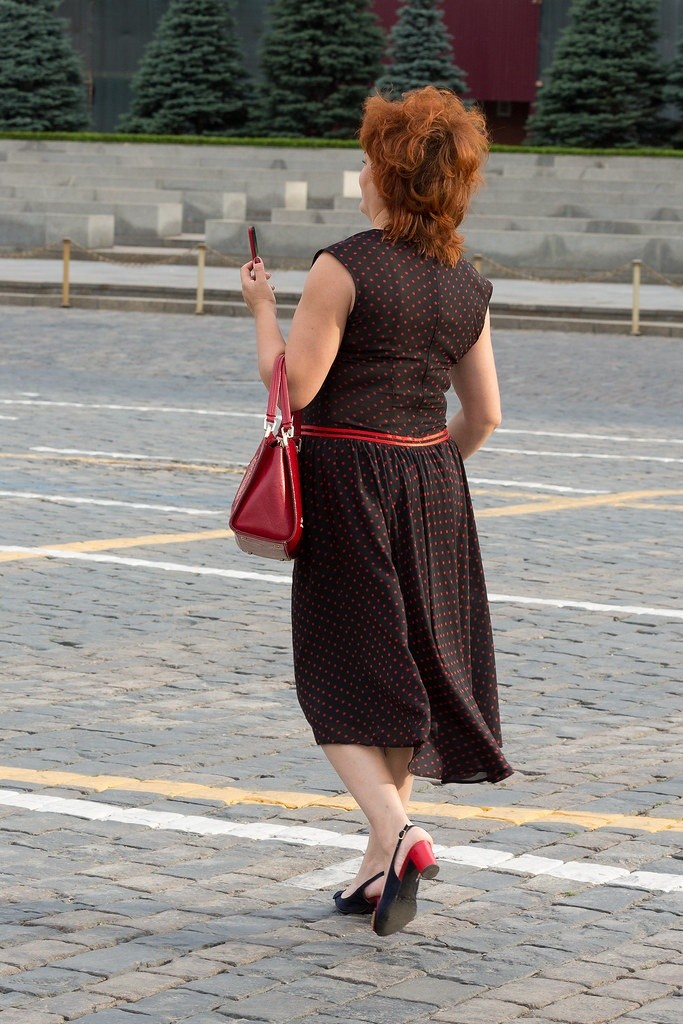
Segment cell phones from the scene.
[246,225,261,264]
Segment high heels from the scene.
[332,869,386,914]
[370,822,440,936]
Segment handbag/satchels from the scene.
[229,353,302,557]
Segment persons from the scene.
[226,87,514,936]
